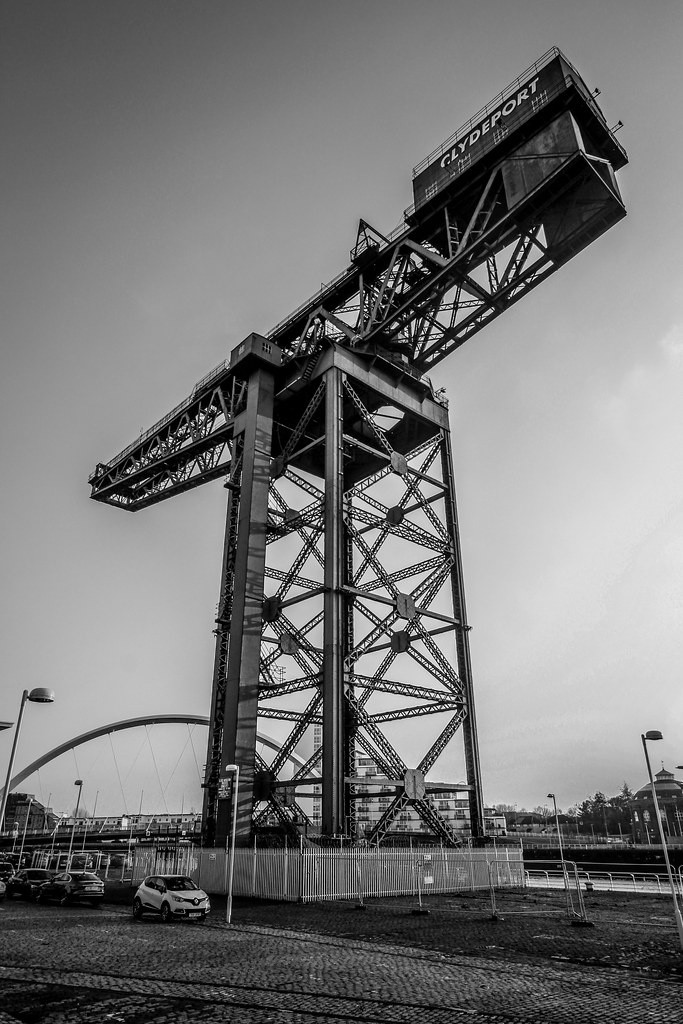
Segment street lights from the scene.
[224,764,239,925]
[8,779,188,882]
[639,729,683,950]
[546,792,570,891]
[0,685,54,841]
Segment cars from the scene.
[0,861,15,888]
[131,874,211,922]
[0,851,198,871]
[37,871,105,910]
[6,868,54,902]
